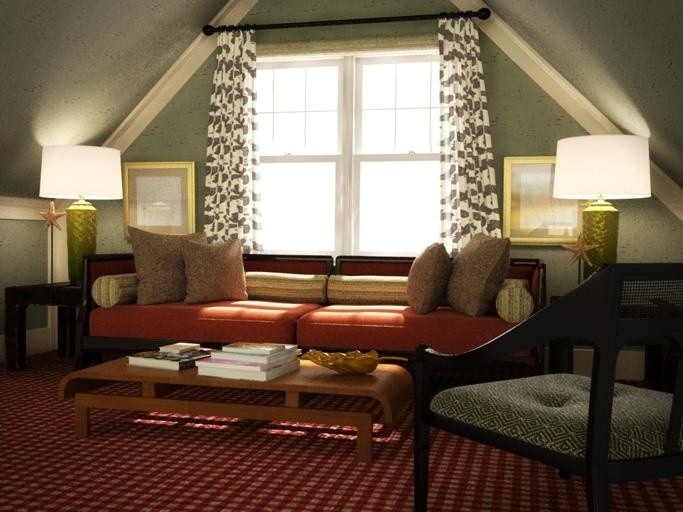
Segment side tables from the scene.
[4,282,86,370]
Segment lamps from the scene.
[38,143,123,281]
[552,133,653,291]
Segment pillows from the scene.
[122,224,252,307]
[404,230,515,318]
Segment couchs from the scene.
[77,251,553,381]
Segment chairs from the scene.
[406,270,680,510]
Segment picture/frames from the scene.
[502,156,585,248]
[121,161,196,239]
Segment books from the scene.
[123,342,220,373]
[195,342,303,384]
